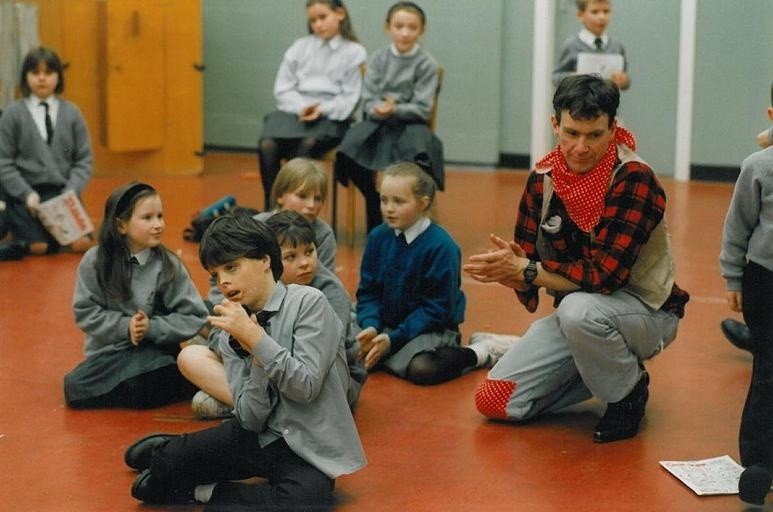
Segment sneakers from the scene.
[190,391,235,417]
[738,464,771,504]
[468,330,522,362]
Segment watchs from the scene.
[522,258,538,291]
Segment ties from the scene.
[40,102,54,145]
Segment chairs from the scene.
[279,64,365,241]
[346,67,443,249]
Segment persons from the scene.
[462,71,690,444]
[64,182,211,408]
[257,0,367,209]
[718,82,773,506]
[208,155,338,306]
[176,210,368,418]
[551,0,631,91]
[0,46,93,262]
[335,1,445,232]
[350,161,524,386]
[124,213,367,512]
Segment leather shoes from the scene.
[720,318,752,354]
[591,384,649,444]
[123,433,180,471]
[131,470,198,506]
